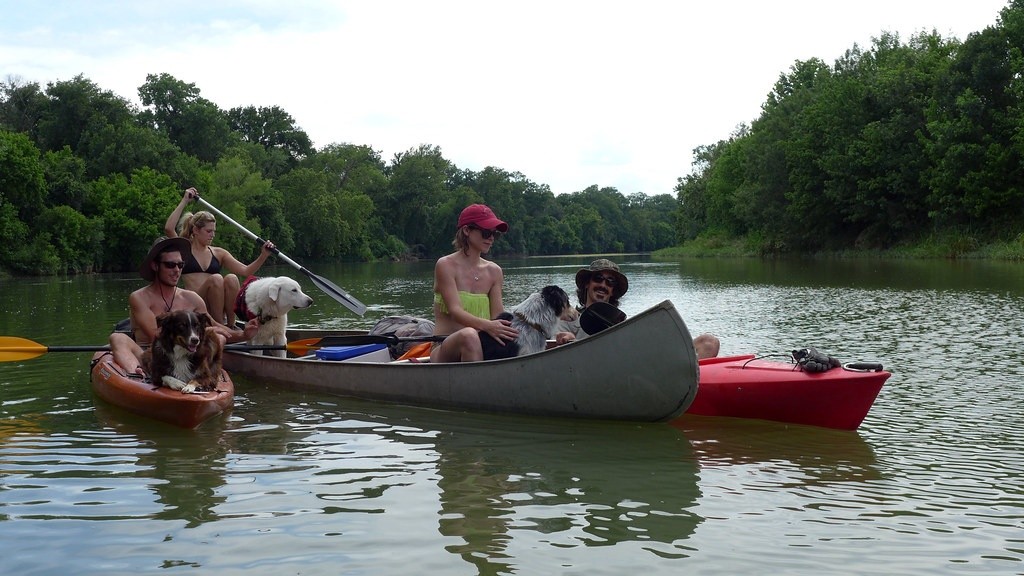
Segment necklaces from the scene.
[465,255,479,280]
[158,281,178,312]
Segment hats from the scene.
[141,236,191,282]
[458,204,508,232]
[576,259,628,300]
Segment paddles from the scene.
[0,336,323,362]
[305,333,447,348]
[188,190,369,317]
[580,303,627,336]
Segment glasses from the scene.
[593,273,618,287]
[158,261,186,269]
[468,226,501,239]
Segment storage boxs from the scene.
[315,342,391,363]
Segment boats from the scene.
[86,339,238,431]
[683,349,892,433]
[220,295,705,423]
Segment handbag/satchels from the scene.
[367,316,435,360]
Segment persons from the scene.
[165,187,278,330]
[556,260,720,361]
[430,204,520,362]
[108,237,261,381]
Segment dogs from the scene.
[478,285,581,361]
[235,275,314,358]
[142,309,223,393]
